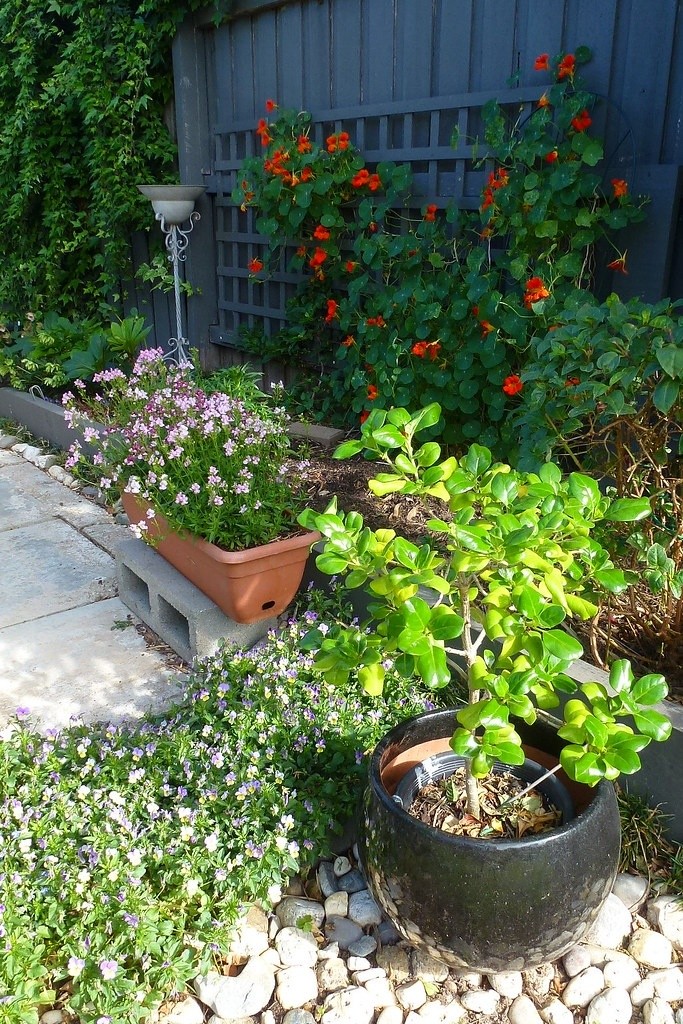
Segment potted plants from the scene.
[297,401,674,973]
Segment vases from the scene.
[117,481,321,626]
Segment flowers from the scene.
[60,344,312,552]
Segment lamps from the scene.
[135,183,210,366]
